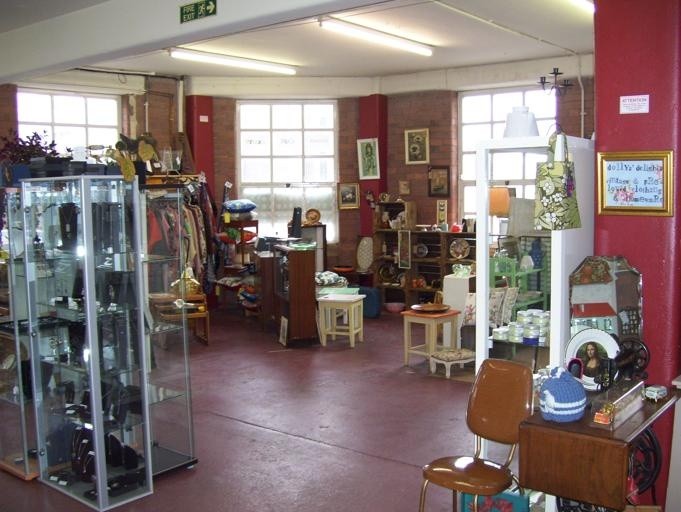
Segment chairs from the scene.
[418,360,533,512]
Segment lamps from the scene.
[489,187,516,238]
[538,68,573,97]
[319,17,437,57]
[166,46,299,77]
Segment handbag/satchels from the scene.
[534,131,582,231]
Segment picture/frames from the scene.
[337,183,360,209]
[428,165,450,197]
[404,128,430,165]
[357,138,380,180]
[595,151,673,216]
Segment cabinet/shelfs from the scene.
[273,244,317,341]
[18,175,154,512]
[373,201,476,316]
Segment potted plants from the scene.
[0,131,74,188]
[115,134,159,184]
[105,145,122,175]
[84,145,104,174]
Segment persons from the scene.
[363,143,375,176]
[582,342,605,377]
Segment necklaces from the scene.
[71,430,80,458]
[56,205,77,234]
[82,454,94,474]
[77,442,87,460]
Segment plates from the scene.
[411,303,450,313]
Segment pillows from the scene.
[217,277,242,287]
[242,285,255,294]
[242,299,263,309]
[240,275,254,286]
[237,263,256,276]
[221,211,259,221]
[216,230,256,244]
[240,291,256,302]
[221,198,257,212]
[315,271,342,285]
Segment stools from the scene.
[429,349,476,379]
[316,294,366,348]
[149,293,210,346]
[318,288,359,328]
[401,310,460,374]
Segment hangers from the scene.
[186,197,191,207]
